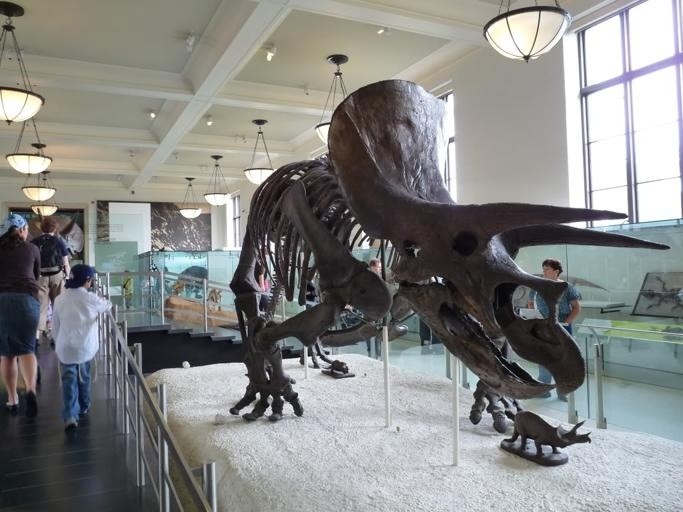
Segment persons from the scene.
[51,263,113,431]
[418,276,446,355]
[370,258,382,276]
[305,273,317,310]
[254,249,270,312]
[527,259,583,401]
[122,270,133,311]
[29,218,72,355]
[0,214,41,417]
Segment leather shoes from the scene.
[25,391,38,419]
[1,400,20,412]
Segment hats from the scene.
[68,262,96,283]
[4,214,27,230]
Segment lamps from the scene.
[266,44,277,62]
[206,115,213,126]
[203,155,232,206]
[483,0,571,63]
[243,118,277,186]
[313,54,348,146]
[0,0,45,125]
[29,203,59,217]
[179,178,202,219]
[20,173,56,201]
[5,116,52,176]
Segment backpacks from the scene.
[34,235,62,278]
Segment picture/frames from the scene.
[630,272,683,319]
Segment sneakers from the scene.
[64,420,78,433]
